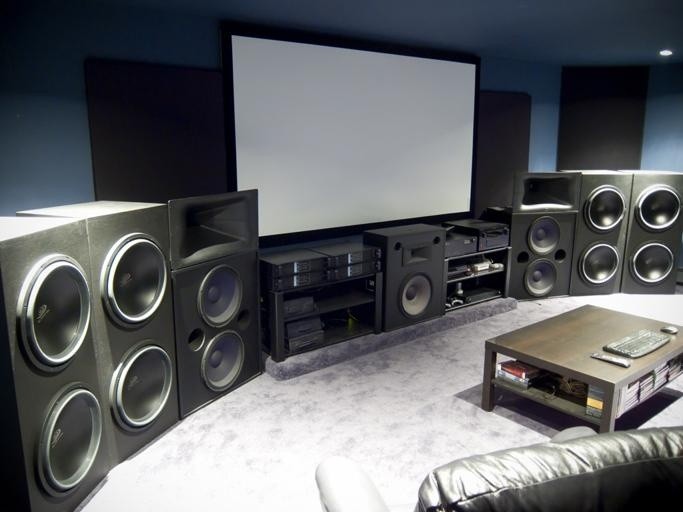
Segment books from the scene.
[497,360,539,389]
[585,359,683,419]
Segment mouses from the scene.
[660,326,679,334]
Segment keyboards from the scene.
[603,328,670,358]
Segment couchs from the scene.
[313,425,683,512]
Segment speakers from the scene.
[363,224,445,333]
[168,188,264,420]
[0,216,110,512]
[561,169,633,296]
[618,170,683,294]
[508,173,581,302]
[17,200,180,471]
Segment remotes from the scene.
[591,353,631,368]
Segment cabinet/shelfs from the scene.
[442,246,512,311]
[262,269,385,363]
